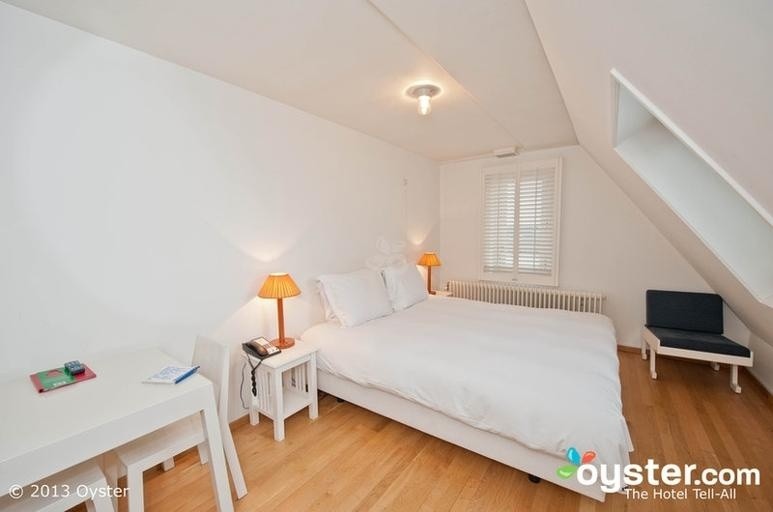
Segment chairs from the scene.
[641,289,755,395]
[104,334,248,512]
[0,460,114,512]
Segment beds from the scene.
[300,296,635,503]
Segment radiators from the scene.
[447,279,607,313]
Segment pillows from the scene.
[314,266,428,327]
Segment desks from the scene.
[0,349,233,511]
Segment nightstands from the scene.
[241,339,320,442]
[430,291,452,296]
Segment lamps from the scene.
[257,272,303,349]
[416,251,443,295]
[402,82,446,118]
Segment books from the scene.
[27,364,96,393]
[140,365,202,384]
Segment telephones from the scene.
[242,336,281,360]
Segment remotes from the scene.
[64,360,85,375]
